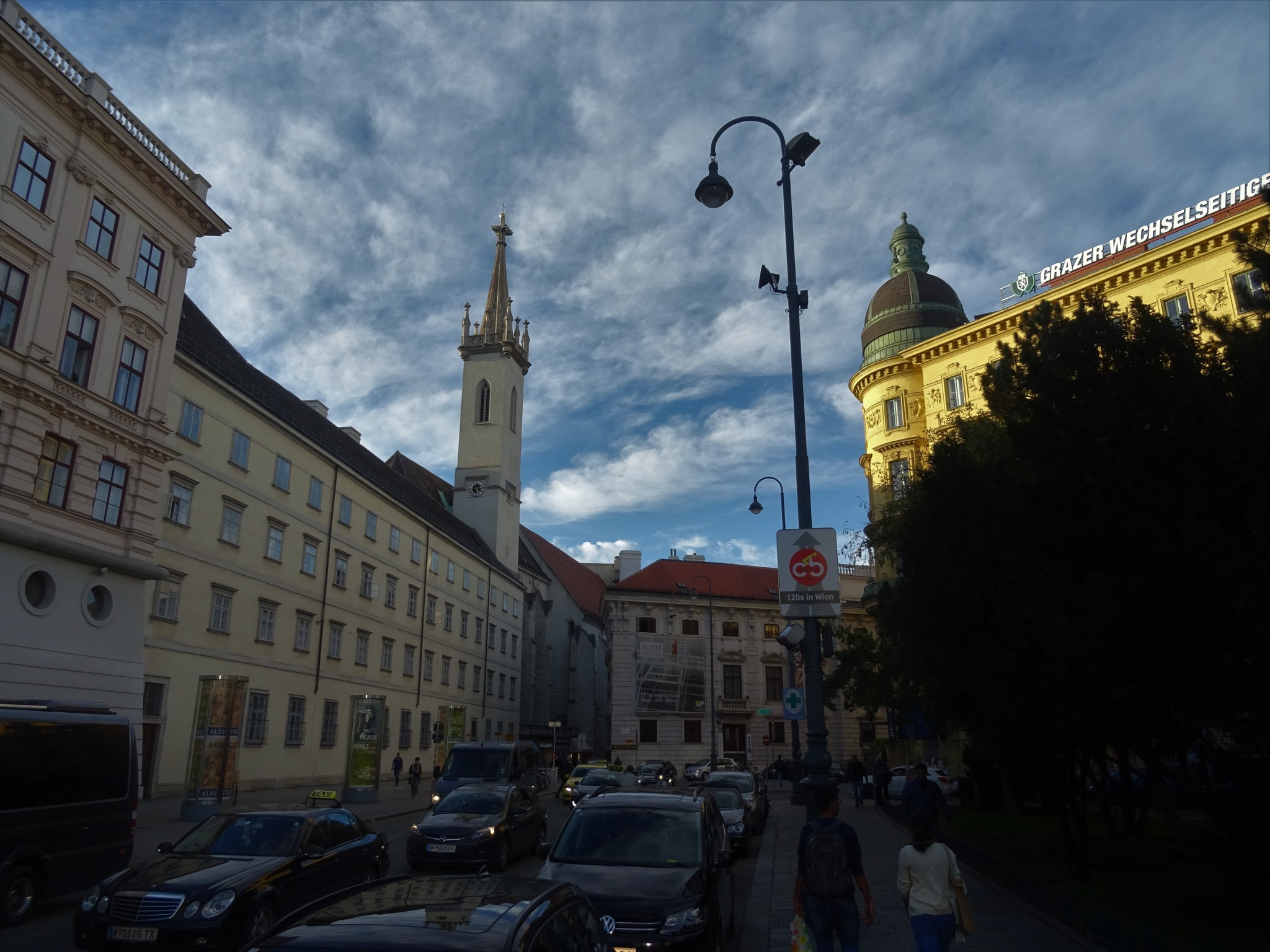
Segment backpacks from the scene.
[804,819,852,898]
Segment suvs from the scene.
[856,765,954,800]
[531,781,737,952]
[683,757,736,780]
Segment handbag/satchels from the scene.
[938,842,976,943]
[789,912,814,952]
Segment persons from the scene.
[409,757,422,799]
[617,765,641,787]
[870,757,892,807]
[656,759,677,787]
[391,753,403,787]
[846,755,869,807]
[555,758,574,799]
[902,763,952,842]
[774,754,785,787]
[794,785,875,952]
[897,807,967,952]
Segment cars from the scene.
[762,759,800,780]
[231,872,610,952]
[691,770,767,856]
[562,758,612,805]
[829,761,845,782]
[569,765,624,811]
[406,781,547,875]
[71,789,391,952]
[622,760,678,786]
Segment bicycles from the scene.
[536,765,551,790]
[746,766,766,784]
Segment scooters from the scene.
[649,770,675,787]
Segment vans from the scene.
[427,738,538,811]
[0,697,137,925]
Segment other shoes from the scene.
[855,803,860,807]
[860,799,863,805]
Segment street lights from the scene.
[746,475,803,810]
[694,115,833,777]
[687,572,718,773]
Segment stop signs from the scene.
[763,735,768,746]
